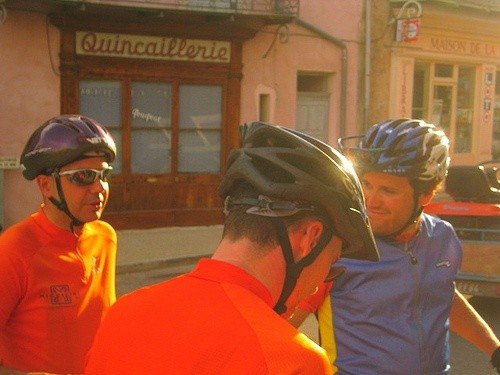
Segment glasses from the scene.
[52,167,118,186]
[338,136,413,167]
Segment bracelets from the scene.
[491,346,500,368]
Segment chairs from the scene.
[445,165,500,204]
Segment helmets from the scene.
[18,115,117,181]
[358,117,452,183]
[216,120,382,263]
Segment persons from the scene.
[0,114,119,375]
[80,119,381,375]
[315,118,500,375]
